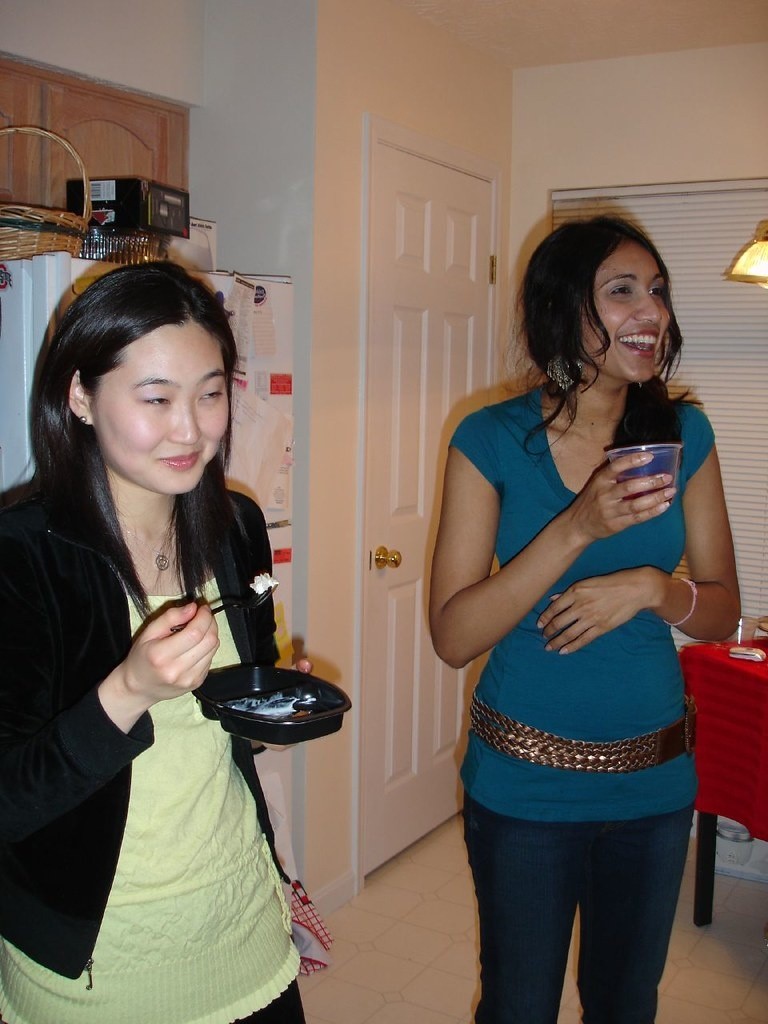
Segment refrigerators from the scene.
[0,251,315,984]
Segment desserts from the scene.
[248,568,279,598]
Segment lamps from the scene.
[720,217,768,294]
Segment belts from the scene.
[469,690,696,774]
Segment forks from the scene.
[169,582,279,633]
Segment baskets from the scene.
[1,126,91,261]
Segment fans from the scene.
[65,175,191,240]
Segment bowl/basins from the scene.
[192,663,352,745]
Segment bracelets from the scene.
[662,576,699,626]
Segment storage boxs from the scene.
[77,224,166,266]
[168,214,218,273]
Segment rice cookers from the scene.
[715,821,757,866]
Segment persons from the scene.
[427,217,744,1024]
[0,262,316,1024]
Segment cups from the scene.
[605,443,683,506]
[734,616,760,648]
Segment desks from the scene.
[678,636,768,928]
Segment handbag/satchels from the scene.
[286,885,337,983]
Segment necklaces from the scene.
[121,525,177,571]
